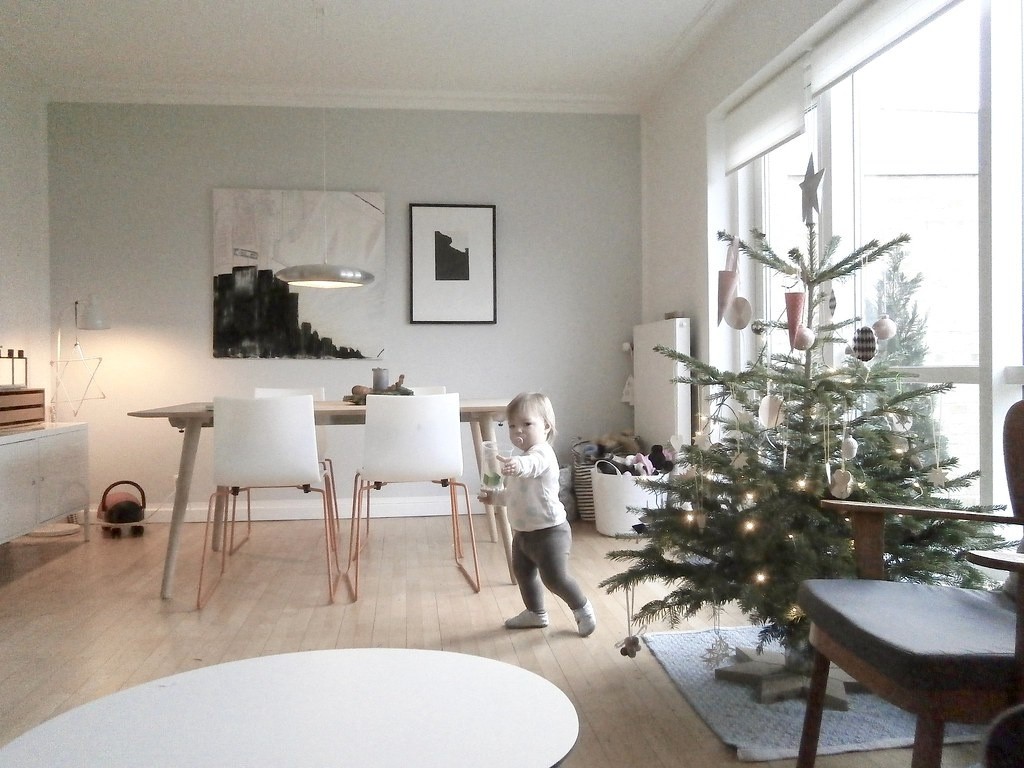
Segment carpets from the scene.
[641,631,983,762]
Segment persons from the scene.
[476,392,595,638]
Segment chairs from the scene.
[795,402,1024,768]
[194,388,341,608]
[347,386,481,604]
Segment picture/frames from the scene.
[410,202,498,325]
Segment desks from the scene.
[0,647,581,768]
[125,400,519,598]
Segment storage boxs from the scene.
[8,350,14,357]
[18,350,24,358]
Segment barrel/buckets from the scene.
[591,460,669,537]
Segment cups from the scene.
[372,368,388,389]
[482,442,514,489]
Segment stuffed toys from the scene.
[586,425,671,477]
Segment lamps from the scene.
[275,96,374,290]
[28,294,111,537]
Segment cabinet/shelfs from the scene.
[0,387,46,429]
[0,421,90,544]
